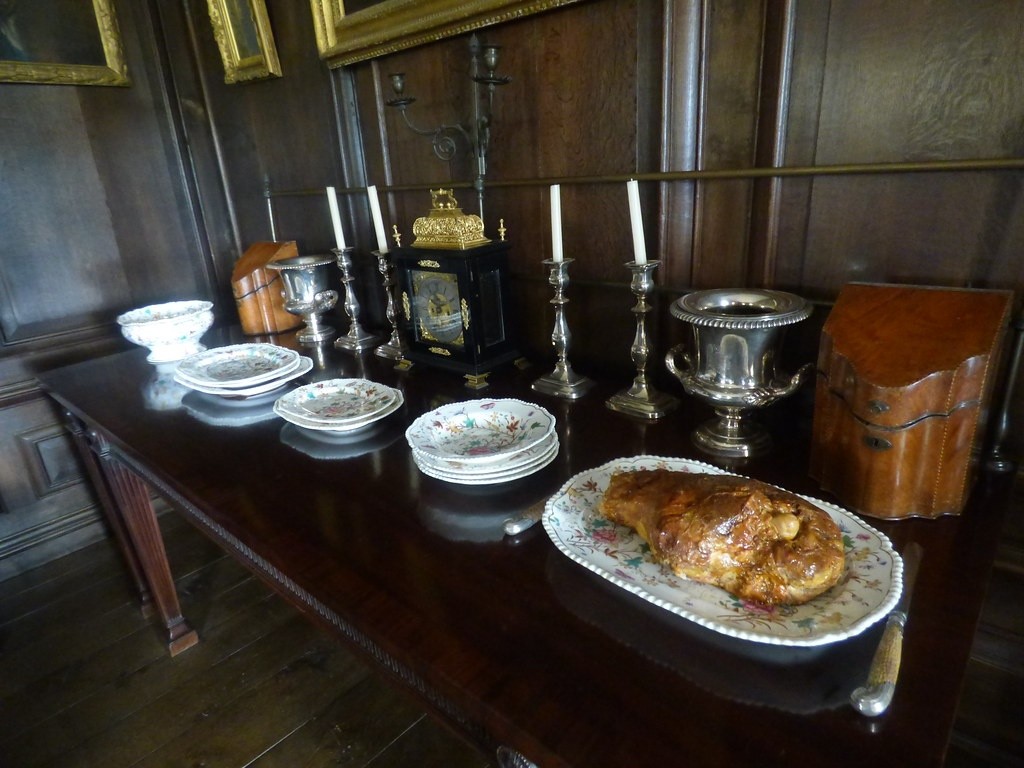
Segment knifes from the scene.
[850,539,924,717]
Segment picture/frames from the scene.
[310,0,580,70]
[206,0,282,85]
[0,0,134,87]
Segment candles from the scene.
[367,184,388,250]
[550,183,563,261]
[626,178,647,264]
[326,186,346,248]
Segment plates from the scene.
[541,453,905,648]
[273,376,405,435]
[406,399,560,485]
[173,341,313,400]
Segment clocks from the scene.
[383,187,533,393]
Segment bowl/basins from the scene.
[116,299,213,364]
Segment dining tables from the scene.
[36,315,1016,767]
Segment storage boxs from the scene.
[812,283,1013,520]
[230,240,303,335]
